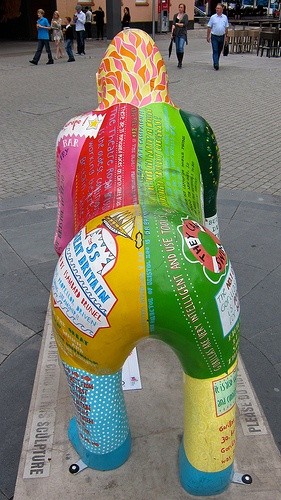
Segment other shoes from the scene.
[214,64,219,70]
[75,53,85,56]
[29,60,38,65]
[87,38,104,40]
[54,53,64,60]
[46,60,53,64]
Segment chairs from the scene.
[226,29,281,58]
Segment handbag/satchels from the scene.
[223,41,229,56]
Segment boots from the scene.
[67,56,76,62]
[176,52,184,68]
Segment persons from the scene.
[206,3,229,71]
[71,5,86,56]
[120,7,131,31]
[84,6,94,40]
[62,16,76,62]
[232,1,241,20]
[92,6,105,40]
[29,8,54,65]
[170,3,189,68]
[50,10,64,60]
